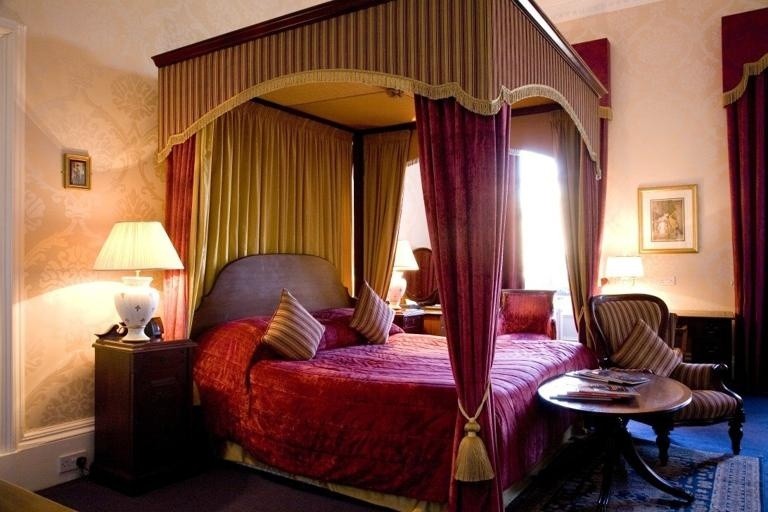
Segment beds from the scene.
[186,253,597,512]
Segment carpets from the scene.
[528,434,763,512]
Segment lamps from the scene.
[388,239,416,309]
[95,219,187,342]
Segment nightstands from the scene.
[386,308,424,335]
[92,338,202,496]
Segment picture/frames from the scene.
[61,152,92,189]
[636,180,699,254]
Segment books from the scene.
[546,381,640,404]
[577,367,649,386]
[564,368,609,383]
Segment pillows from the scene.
[612,317,682,376]
[349,280,398,343]
[258,286,328,360]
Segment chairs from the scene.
[496,289,559,342]
[584,293,749,462]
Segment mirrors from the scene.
[402,245,437,304]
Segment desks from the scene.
[537,368,692,512]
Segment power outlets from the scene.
[58,449,88,474]
